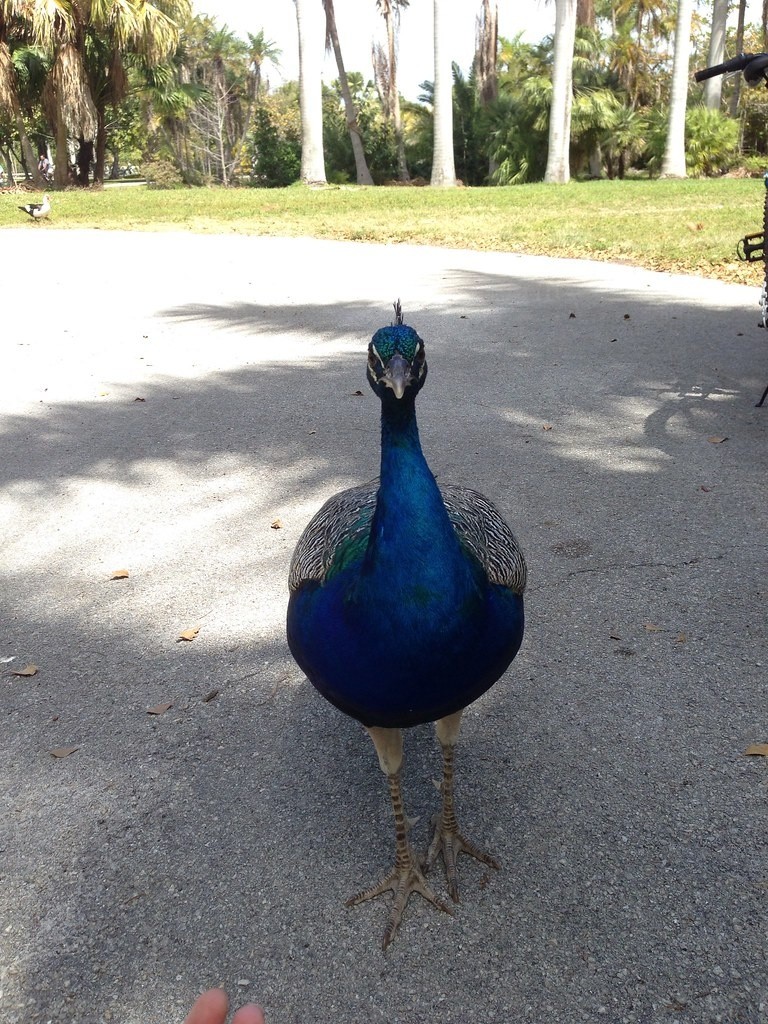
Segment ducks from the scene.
[19,193,52,222]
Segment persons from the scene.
[37,154,56,187]
[0,164,4,193]
[123,162,134,177]
[179,986,268,1024]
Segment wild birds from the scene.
[284,297,526,954]
